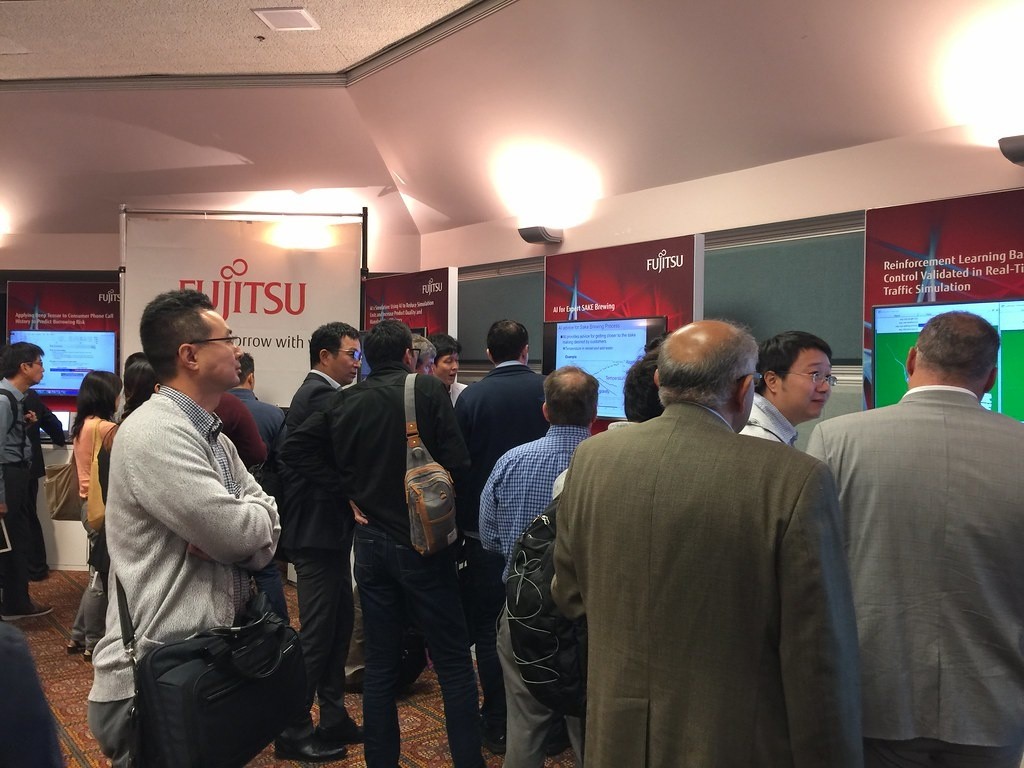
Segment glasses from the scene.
[404,349,422,357]
[330,348,363,365]
[737,373,762,386]
[176,336,242,356]
[775,371,840,386]
[17,362,43,368]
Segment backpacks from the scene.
[506,492,588,719]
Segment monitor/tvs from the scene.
[871,297,1023,424]
[9,329,117,397]
[357,326,427,383]
[542,316,668,421]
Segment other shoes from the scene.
[1,604,54,621]
[84,650,93,661]
[344,669,365,691]
[67,641,86,653]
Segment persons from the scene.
[277,321,362,761]
[455,319,570,754]
[0,341,53,621]
[805,313,1024,768]
[22,389,66,581]
[551,353,665,502]
[479,368,599,767]
[226,352,289,624]
[426,332,469,409]
[87,288,282,768]
[550,321,861,768]
[740,331,838,447]
[68,369,123,660]
[88,359,160,595]
[410,334,435,376]
[282,321,484,768]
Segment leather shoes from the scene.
[275,733,348,763]
[546,729,569,754]
[479,716,507,754]
[316,716,365,744]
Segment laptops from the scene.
[39,411,69,439]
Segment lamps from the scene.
[998,135,1024,166]
[517,224,563,244]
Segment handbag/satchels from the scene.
[87,419,105,531]
[133,592,307,768]
[248,456,314,563]
[43,450,82,521]
[404,434,458,557]
